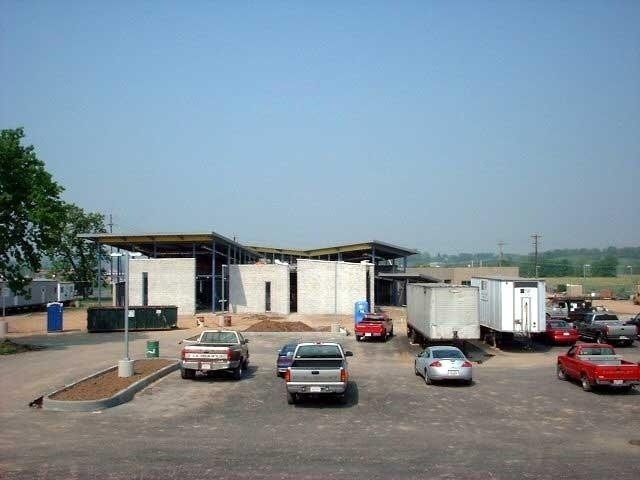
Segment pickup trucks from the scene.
[286,342,353,402]
[181,330,249,379]
[546,320,578,345]
[558,343,640,392]
[578,310,637,346]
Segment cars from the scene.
[276,344,297,377]
[356,313,393,341]
[414,346,473,385]
[625,313,640,341]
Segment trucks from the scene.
[545,300,608,318]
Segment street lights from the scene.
[111,250,142,380]
